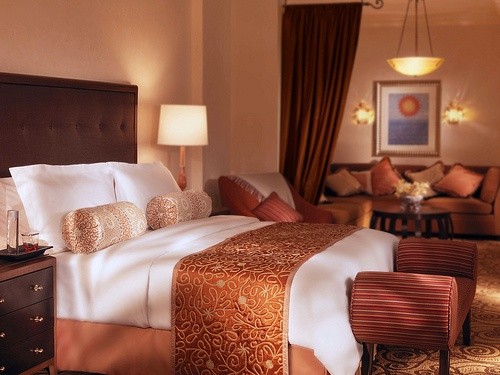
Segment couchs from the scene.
[318,160,500,238]
[218,170,337,224]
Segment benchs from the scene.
[348,237,480,375]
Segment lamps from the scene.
[386,0,445,78]
[351,99,375,125]
[158,104,210,191]
[445,100,465,125]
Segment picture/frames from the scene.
[371,79,443,158]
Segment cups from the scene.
[6,210,19,255]
[22,232,39,252]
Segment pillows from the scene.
[432,162,484,199]
[368,154,407,196]
[350,169,373,195]
[326,164,363,198]
[403,159,447,198]
[8,160,214,255]
[251,190,305,223]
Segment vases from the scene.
[400,195,425,207]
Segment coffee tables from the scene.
[371,207,456,239]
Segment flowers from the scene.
[389,179,430,199]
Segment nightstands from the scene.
[212,203,233,216]
[0,253,59,375]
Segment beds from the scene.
[0,71,401,375]
